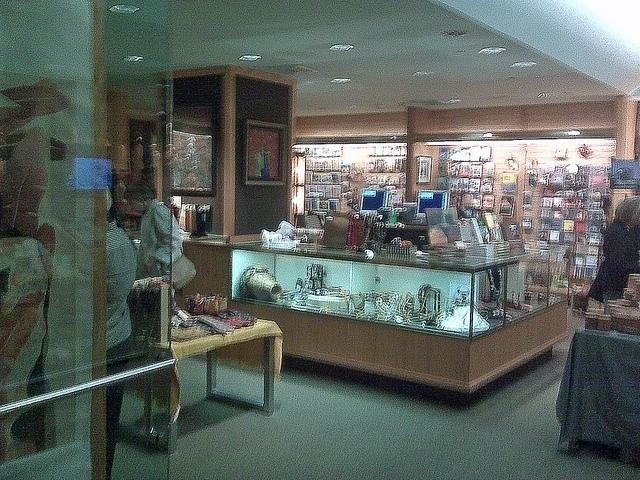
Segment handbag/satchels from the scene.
[160,251,197,291]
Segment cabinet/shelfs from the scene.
[229,246,571,400]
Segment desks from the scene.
[124,316,283,450]
[558,329,640,464]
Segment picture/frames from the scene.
[243,118,291,190]
[167,95,220,197]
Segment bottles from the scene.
[185,203,208,237]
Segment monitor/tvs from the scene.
[416,189,450,215]
[69,157,113,190]
[359,188,388,211]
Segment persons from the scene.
[124,179,196,291]
[105,210,138,480]
[587,196,640,310]
